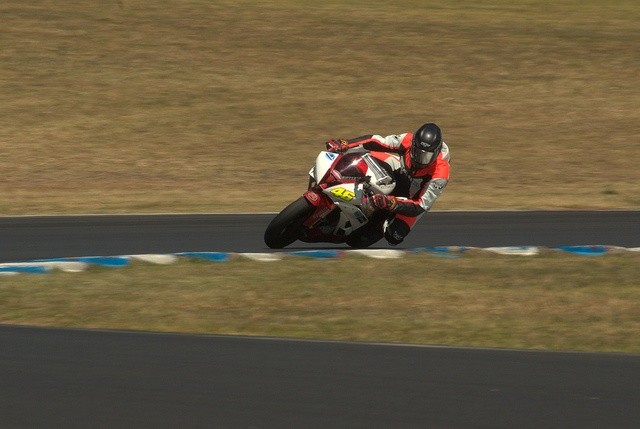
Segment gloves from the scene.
[326,138,350,152]
[371,194,398,212]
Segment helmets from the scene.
[410,123,442,169]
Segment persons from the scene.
[323,121,452,247]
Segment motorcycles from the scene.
[264,143,410,249]
